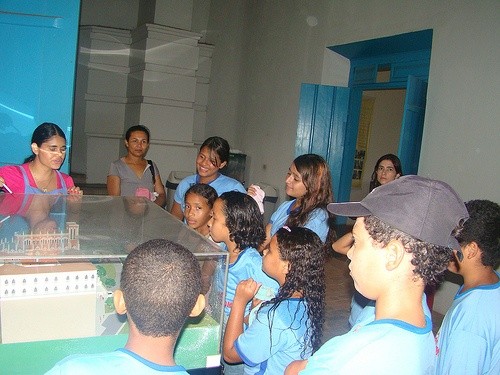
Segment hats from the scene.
[327,176,471,262]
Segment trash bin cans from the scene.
[255,181,279,227]
[165,171,194,213]
